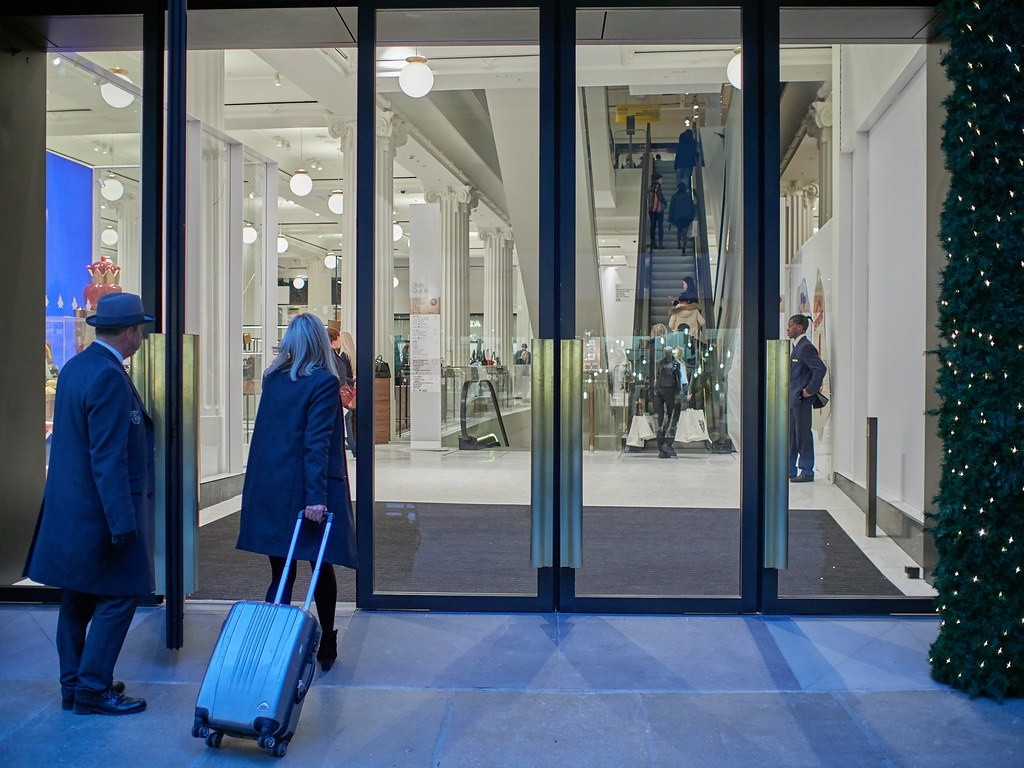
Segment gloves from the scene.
[112,530,138,552]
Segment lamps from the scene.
[325,233,339,268]
[290,124,311,197]
[726,46,742,89]
[293,264,304,289]
[683,103,699,126]
[100,51,134,108]
[328,176,343,215]
[393,208,403,243]
[100,137,124,200]
[393,275,399,288]
[273,72,282,87]
[242,195,257,244]
[399,44,433,98]
[250,191,256,198]
[277,223,288,253]
[311,160,323,170]
[96,179,106,188]
[102,204,117,245]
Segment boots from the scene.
[316,629,339,671]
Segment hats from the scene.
[87,293,155,329]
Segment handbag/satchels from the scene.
[626,403,657,448]
[374,355,391,378]
[339,384,356,407]
[652,184,662,212]
[674,399,709,444]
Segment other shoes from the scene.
[662,444,677,457]
[652,245,657,248]
[658,245,665,249]
[683,252,687,256]
[658,451,670,458]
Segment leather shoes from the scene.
[790,473,813,482]
[73,688,147,715]
[62,680,125,710]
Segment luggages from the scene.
[191,510,335,757]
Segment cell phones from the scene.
[669,296,672,299]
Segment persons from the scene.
[636,275,706,459]
[515,343,532,364]
[648,174,667,249]
[234,314,361,673]
[22,291,155,714]
[620,154,662,169]
[674,129,697,195]
[786,315,828,482]
[667,182,696,255]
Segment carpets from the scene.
[185,505,905,601]
